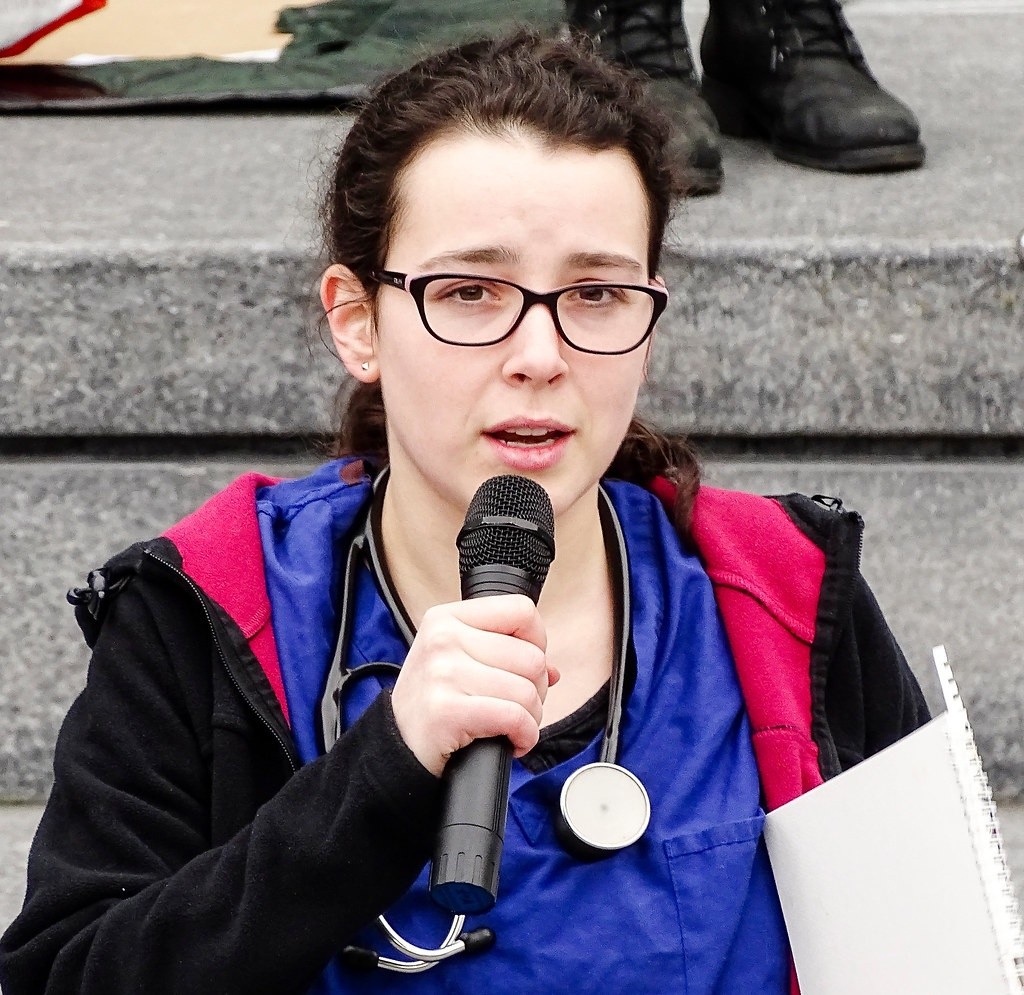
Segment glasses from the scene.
[361,262,670,357]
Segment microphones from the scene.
[428,474,556,916]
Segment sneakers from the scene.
[701,1,925,170]
[567,0,723,192]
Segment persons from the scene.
[0,33,939,993]
[566,2,935,197]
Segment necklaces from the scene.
[361,461,653,850]
